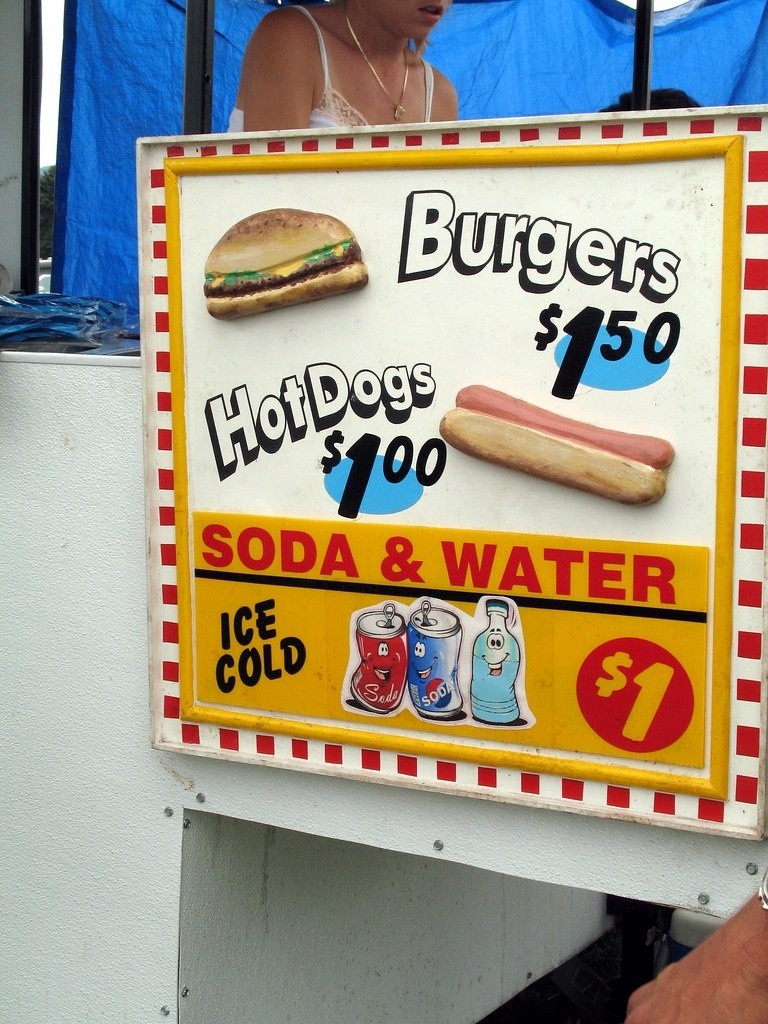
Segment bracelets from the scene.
[758,869,768,909]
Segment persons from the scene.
[224,0,458,133]
[625,867,768,1024]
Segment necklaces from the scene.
[344,7,410,121]
